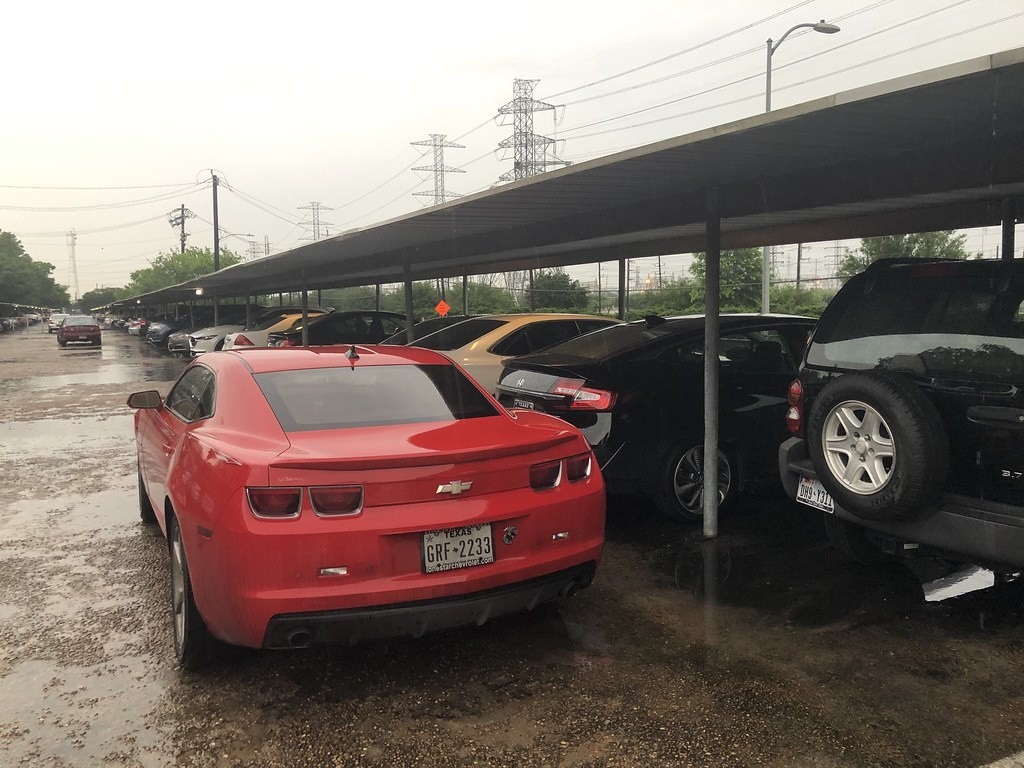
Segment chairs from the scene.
[749,341,785,374]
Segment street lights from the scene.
[759,18,841,316]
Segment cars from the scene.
[0,308,51,333]
[402,313,729,399]
[56,315,102,347]
[494,310,820,524]
[90,302,337,358]
[378,314,482,346]
[49,313,71,333]
[267,310,420,346]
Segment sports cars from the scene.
[124,344,609,669]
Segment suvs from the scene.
[777,251,1023,579]
